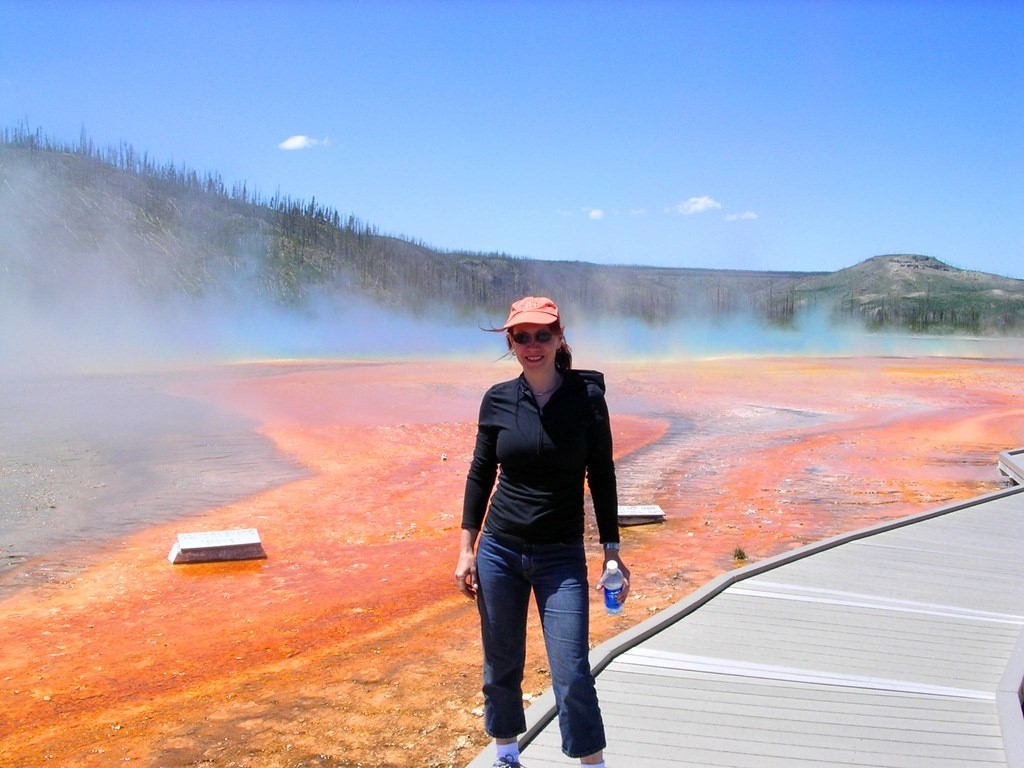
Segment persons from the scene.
[453,296,632,768]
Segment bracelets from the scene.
[605,542,620,551]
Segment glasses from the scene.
[512,332,557,343]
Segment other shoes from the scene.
[493,754,525,768]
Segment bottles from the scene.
[603,559,622,616]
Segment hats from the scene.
[504,297,559,329]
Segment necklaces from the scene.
[533,376,561,396]
